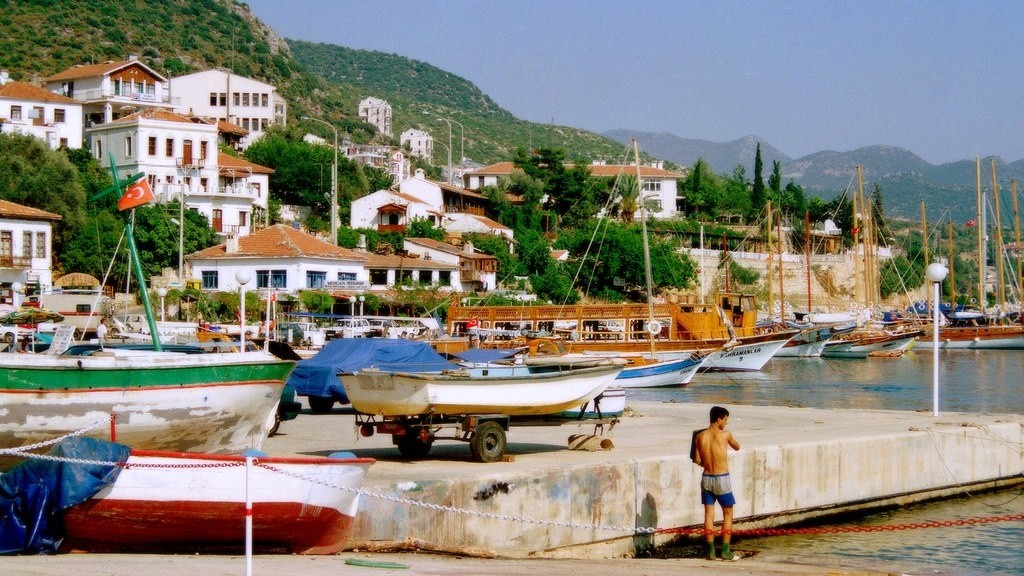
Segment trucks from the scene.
[23,290,112,341]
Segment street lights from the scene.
[437,118,464,188]
[925,262,947,417]
[235,269,251,352]
[300,115,338,246]
[11,282,23,344]
[158,288,168,345]
[423,111,452,185]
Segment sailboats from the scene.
[526,343,742,390]
[1,148,299,458]
[440,136,806,373]
[680,155,1024,358]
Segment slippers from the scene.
[722,555,739,561]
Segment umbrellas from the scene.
[0,306,65,354]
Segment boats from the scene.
[61,412,377,555]
[538,388,625,420]
[334,364,627,416]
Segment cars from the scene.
[331,320,370,340]
[117,313,147,333]
[369,319,407,339]
[108,315,123,340]
[59,344,303,437]
[288,322,324,346]
[1,311,52,344]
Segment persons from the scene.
[10,337,30,353]
[97,319,109,343]
[693,406,740,562]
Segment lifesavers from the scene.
[919,304,925,310]
[1016,300,1021,305]
[918,299,925,304]
[931,303,934,311]
[850,300,882,318]
[992,303,1000,310]
[647,321,662,334]
[982,301,988,307]
[945,302,951,308]
[971,298,977,303]
[955,303,958,308]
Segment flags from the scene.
[117,178,156,211]
[966,218,975,226]
[851,226,860,235]
[466,316,478,328]
